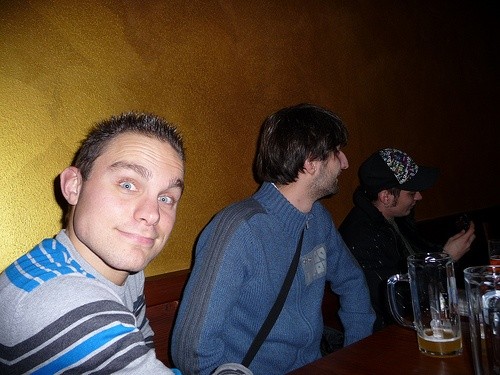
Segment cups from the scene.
[463,265,500,375]
[387,252,463,358]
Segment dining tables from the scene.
[286,288,491,375]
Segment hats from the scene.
[358,148,434,193]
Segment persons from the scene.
[321,148,476,357]
[171,104,376,375]
[0,112,253,375]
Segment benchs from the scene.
[141,210,488,369]
